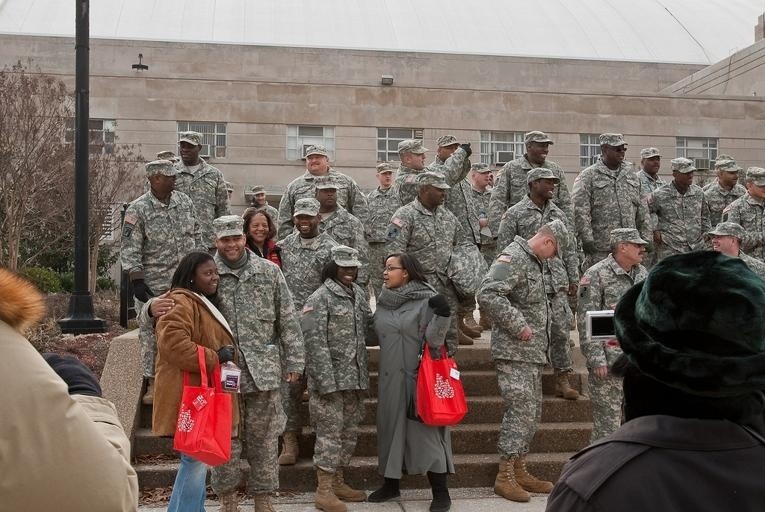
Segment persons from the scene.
[475,220,569,500]
[367,131,581,345]
[278,144,372,250]
[0,267,140,512]
[118,131,233,328]
[241,185,279,245]
[301,246,373,512]
[574,229,648,445]
[368,255,455,512]
[545,252,765,510]
[136,214,305,512]
[277,196,342,468]
[243,210,282,269]
[293,176,370,291]
[151,251,243,512]
[572,132,765,282]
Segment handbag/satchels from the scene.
[172,345,233,465]
[418,334,469,426]
[407,333,448,421]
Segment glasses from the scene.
[384,267,400,272]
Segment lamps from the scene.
[381,74,394,85]
[131,53,149,74]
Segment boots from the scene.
[554,371,579,399]
[278,431,300,465]
[514,457,554,493]
[314,470,347,512]
[494,458,531,502]
[254,494,276,512]
[217,491,239,512]
[427,471,452,512]
[368,478,400,502]
[459,307,491,345]
[333,466,367,502]
[142,378,154,405]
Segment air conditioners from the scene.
[494,150,516,165]
[692,158,712,171]
[301,143,316,159]
[198,144,211,157]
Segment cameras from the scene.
[586,310,616,341]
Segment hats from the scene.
[212,214,245,239]
[225,182,233,192]
[613,250,764,397]
[471,163,491,174]
[145,131,204,178]
[291,145,340,218]
[330,244,362,268]
[527,168,560,185]
[398,135,460,191]
[600,134,697,175]
[708,155,765,240]
[376,163,393,174]
[524,131,553,148]
[252,185,265,195]
[610,228,649,248]
[546,219,570,260]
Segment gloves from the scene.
[47,354,102,397]
[459,143,471,158]
[131,278,154,303]
[216,344,235,364]
[429,294,450,316]
